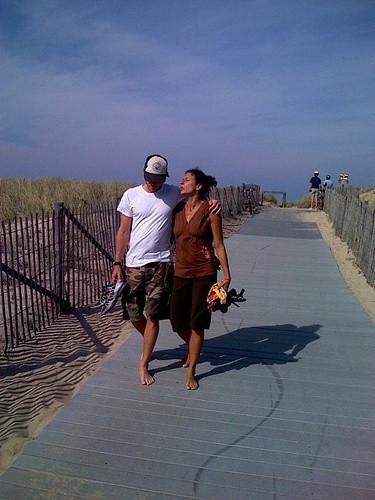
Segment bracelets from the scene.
[112,261,120,265]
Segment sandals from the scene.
[90,279,127,315]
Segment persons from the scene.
[111,153,222,386]
[323,175,333,189]
[309,171,321,210]
[168,169,230,390]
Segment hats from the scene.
[143,154,168,183]
[313,170,319,174]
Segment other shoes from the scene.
[208,288,246,314]
